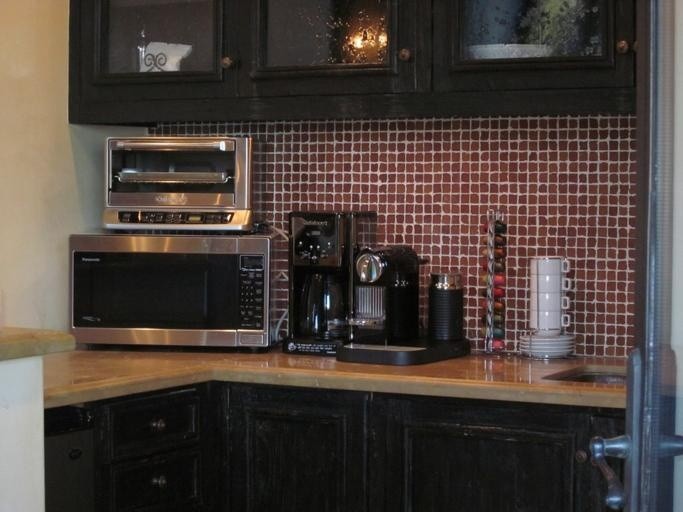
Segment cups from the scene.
[528,256,574,334]
[430,272,464,341]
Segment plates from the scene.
[467,44,556,60]
[520,333,576,360]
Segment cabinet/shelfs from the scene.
[43,381,670,512]
[68,0,636,123]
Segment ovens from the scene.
[102,133,255,231]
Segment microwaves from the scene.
[67,234,271,349]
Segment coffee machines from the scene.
[287,210,377,357]
